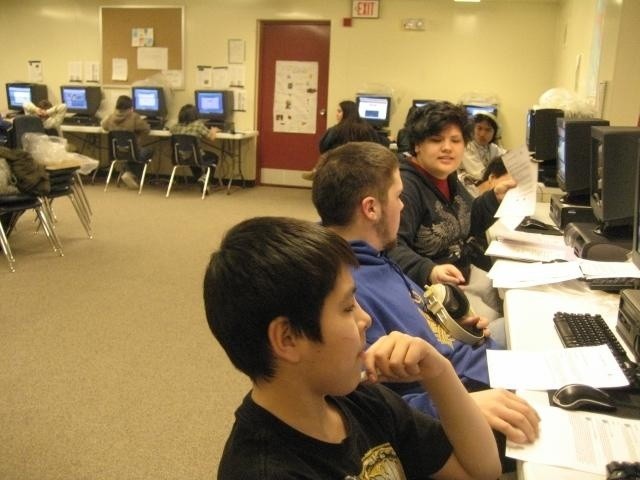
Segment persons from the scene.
[309,100,540,444]
[199,216,505,479]
[100,94,155,191]
[169,104,220,194]
[22,100,69,139]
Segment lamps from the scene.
[401,17,427,30]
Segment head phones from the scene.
[423,281,485,345]
[473,110,502,140]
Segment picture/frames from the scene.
[99,4,185,90]
[598,81,607,119]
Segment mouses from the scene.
[522,219,547,230]
[553,383,617,413]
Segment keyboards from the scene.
[553,311,640,392]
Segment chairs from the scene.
[0,115,93,272]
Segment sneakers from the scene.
[198,175,211,195]
[122,172,139,191]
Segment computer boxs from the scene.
[563,222,633,262]
[550,194,596,229]
[615,288,640,364]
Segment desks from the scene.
[485,182,621,301]
[502,289,640,480]
[2,118,259,199]
[389,143,398,150]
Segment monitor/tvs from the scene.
[355,92,497,128]
[556,117,610,205]
[526,108,564,162]
[6,83,235,134]
[589,125,640,271]
